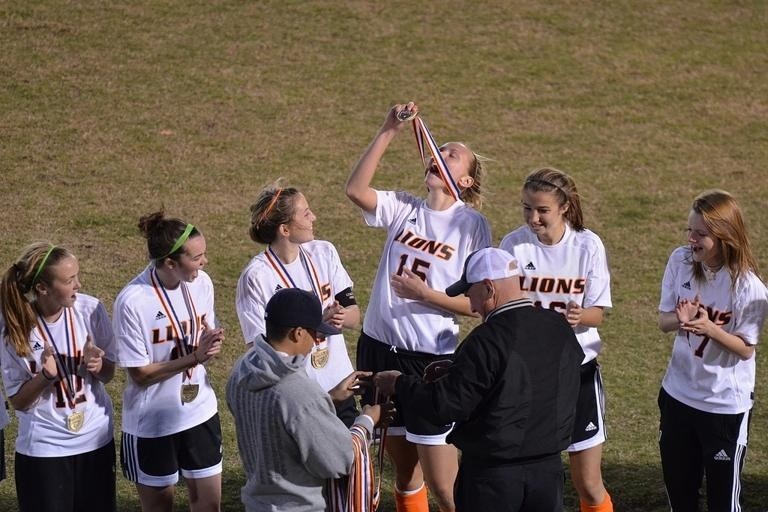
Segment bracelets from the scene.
[41,368,55,380]
[193,353,199,365]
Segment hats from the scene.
[444,245,524,298]
[264,287,341,338]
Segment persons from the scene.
[372,247,583,511]
[113,213,229,510]
[492,167,613,510]
[226,288,393,512]
[234,187,367,432]
[0,241,119,511]
[347,103,497,511]
[0,387,9,482]
[653,185,767,511]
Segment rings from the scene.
[692,328,694,332]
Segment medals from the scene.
[395,109,417,121]
[181,384,198,402]
[67,411,84,432]
[312,348,329,369]
